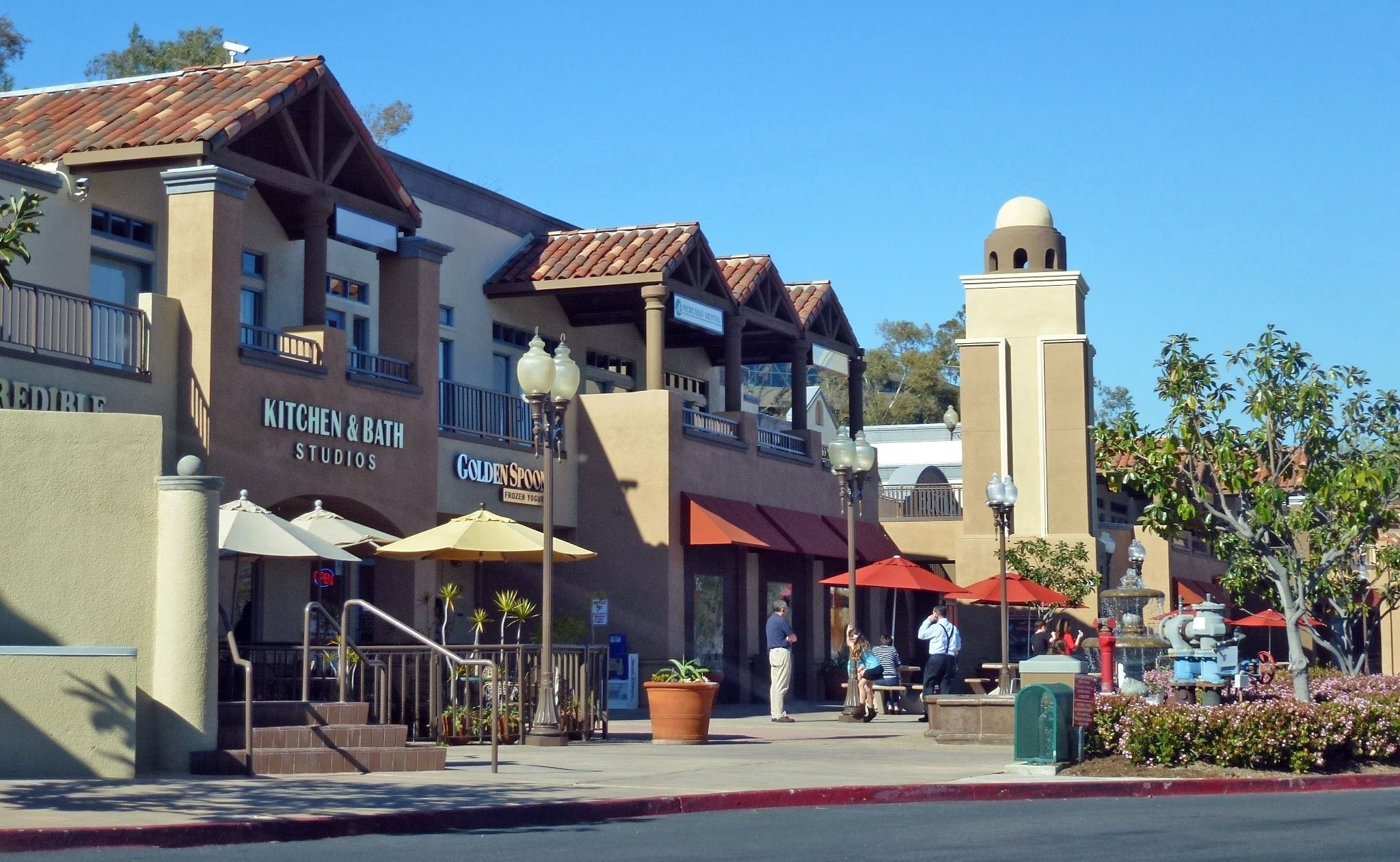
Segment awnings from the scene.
[1170,576,1267,609]
[679,490,906,559]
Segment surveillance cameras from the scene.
[75,177,89,195]
[223,41,250,63]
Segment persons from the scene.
[916,604,962,722]
[845,623,884,723]
[1030,619,1057,658]
[874,635,905,715]
[1049,617,1084,656]
[1046,638,1069,656]
[765,600,798,722]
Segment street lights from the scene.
[985,472,1019,695]
[1098,531,1116,591]
[1128,539,1146,579]
[517,325,580,745]
[828,423,875,724]
[1354,553,1370,675]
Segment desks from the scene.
[982,662,1018,695]
[896,664,921,683]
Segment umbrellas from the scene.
[1230,608,1328,663]
[214,485,602,644]
[946,571,1091,693]
[818,553,973,681]
[1148,603,1235,632]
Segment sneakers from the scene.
[771,701,951,725]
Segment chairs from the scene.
[481,661,517,710]
[483,665,540,728]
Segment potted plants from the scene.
[431,580,493,746]
[558,684,602,742]
[642,654,720,745]
[483,587,538,743]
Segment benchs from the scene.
[902,682,924,714]
[842,682,904,713]
[965,678,990,694]
